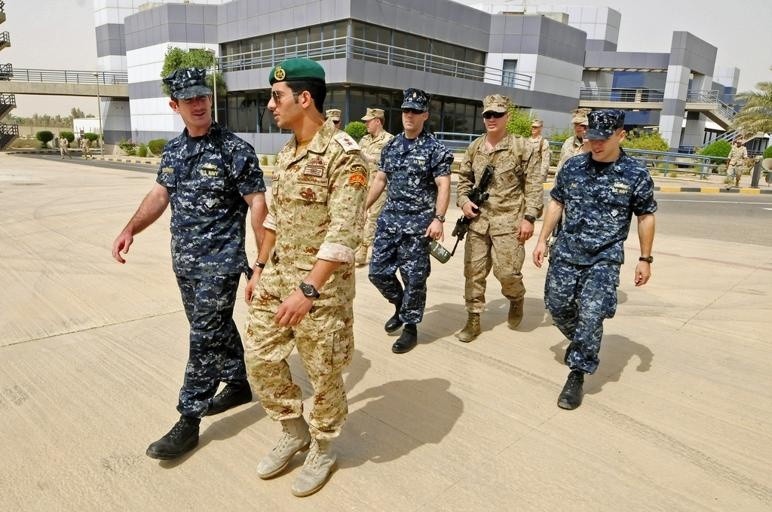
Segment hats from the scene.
[583,109,625,140]
[269,58,325,85]
[162,67,214,101]
[401,88,433,112]
[361,107,385,121]
[326,108,342,123]
[481,94,511,115]
[571,108,592,126]
[531,119,543,127]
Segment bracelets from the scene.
[255,261,265,268]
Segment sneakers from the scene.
[558,371,584,410]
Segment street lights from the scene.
[93,72,104,155]
[205,48,220,124]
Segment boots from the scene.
[508,298,524,330]
[205,381,253,416]
[146,417,201,460]
[458,313,481,342]
[385,301,404,332]
[256,415,311,479]
[292,439,338,497]
[393,324,417,353]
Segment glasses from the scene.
[483,112,506,119]
[272,91,302,106]
[402,108,423,114]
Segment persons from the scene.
[532,109,658,410]
[245,57,370,496]
[59,135,72,159]
[80,135,91,160]
[456,93,544,342]
[325,108,343,129]
[527,120,549,183]
[540,107,593,257]
[357,107,396,263]
[726,140,748,186]
[365,87,452,353]
[112,65,268,459]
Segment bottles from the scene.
[421,236,451,264]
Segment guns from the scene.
[451,165,495,257]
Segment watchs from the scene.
[639,256,653,263]
[434,215,445,222]
[299,282,320,299]
[525,215,535,223]
